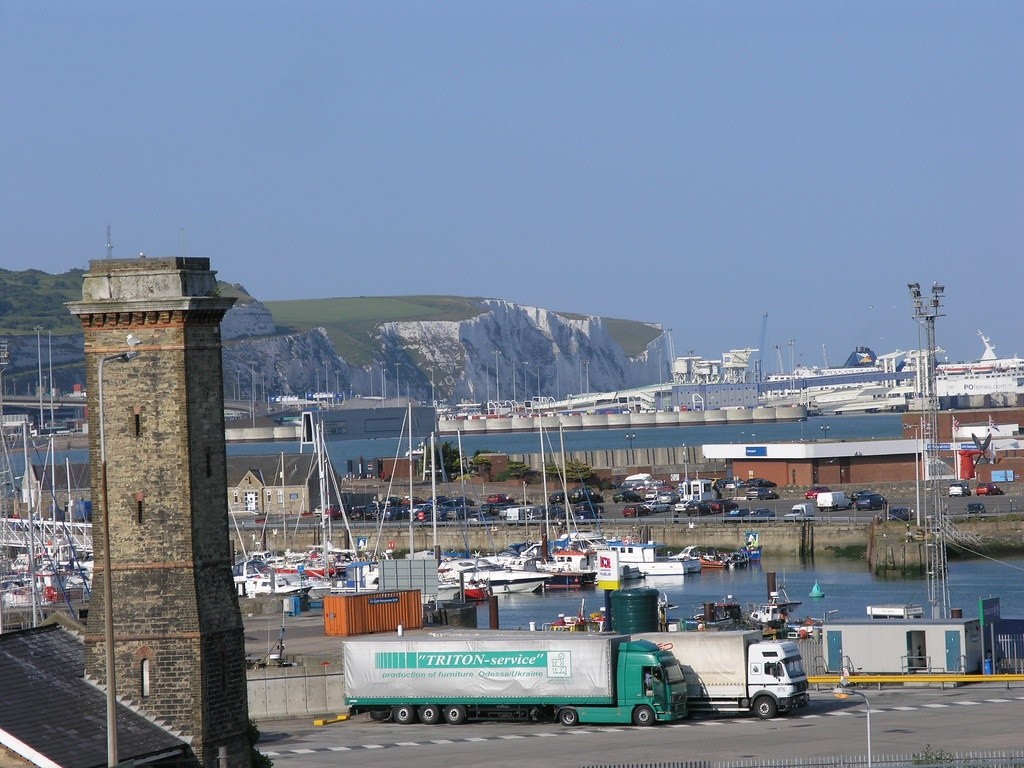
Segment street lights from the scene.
[821,424,830,439]
[833,688,866,768]
[98,351,140,768]
[625,432,636,449]
[903,422,922,527]
[523,481,528,535]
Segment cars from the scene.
[568,487,603,503]
[480,504,503,517]
[534,506,570,520]
[325,508,341,519]
[746,487,776,500]
[716,479,740,488]
[401,496,426,505]
[468,513,493,526]
[383,507,409,520]
[645,490,658,499]
[623,504,650,518]
[351,505,366,521]
[426,496,449,505]
[948,482,971,496]
[365,503,384,520]
[976,482,1001,496]
[380,497,402,507]
[612,490,641,503]
[487,493,514,504]
[449,496,474,508]
[418,505,449,521]
[964,503,986,514]
[746,478,777,488]
[549,492,570,504]
[744,508,775,524]
[649,483,674,491]
[710,478,721,488]
[658,491,679,504]
[686,502,713,517]
[499,506,512,517]
[709,498,739,514]
[314,504,333,514]
[728,508,749,517]
[409,503,432,513]
[805,486,832,500]
[642,500,670,514]
[851,490,871,502]
[886,506,912,521]
[674,499,698,513]
[856,493,887,511]
[574,502,604,514]
[572,511,603,524]
[446,506,476,520]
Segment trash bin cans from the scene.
[951,608,962,618]
[288,595,301,616]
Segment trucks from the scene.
[817,491,852,511]
[506,506,542,524]
[784,503,814,522]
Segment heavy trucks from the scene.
[617,630,809,720]
[343,634,688,727]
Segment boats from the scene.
[746,568,839,636]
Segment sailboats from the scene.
[1,423,94,606]
[234,403,747,597]
